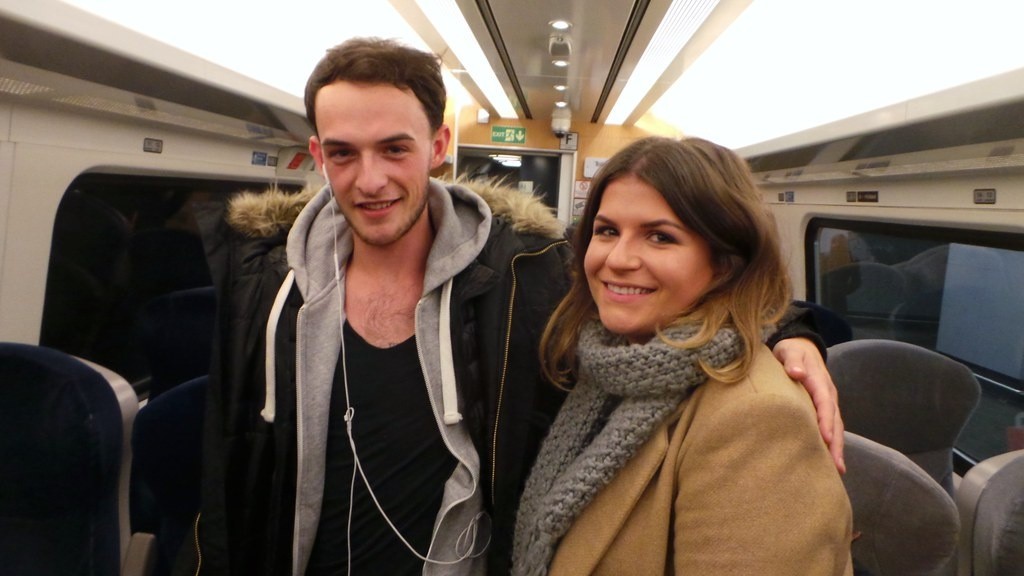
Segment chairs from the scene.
[957,449,1024,576]
[840,429,963,576]
[826,337,983,500]
[0,342,139,576]
[123,373,210,576]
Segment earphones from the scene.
[322,163,331,185]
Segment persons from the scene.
[175,37,854,576]
[819,234,862,317]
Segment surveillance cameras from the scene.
[551,119,571,138]
